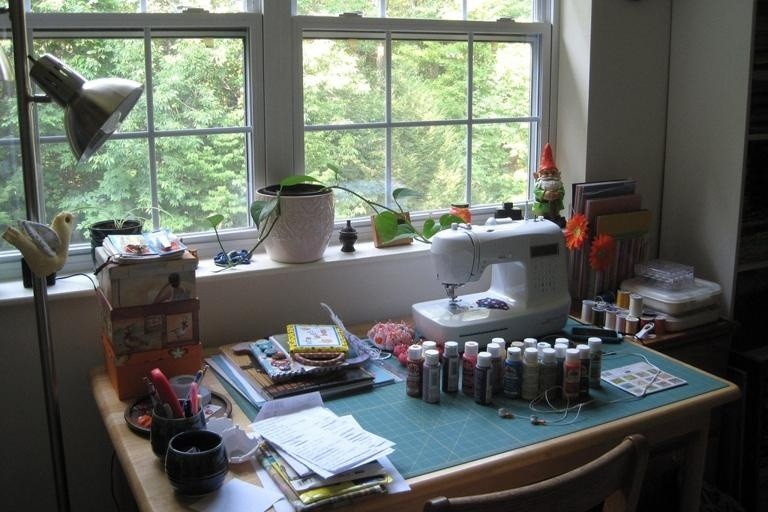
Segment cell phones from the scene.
[571,327,619,344]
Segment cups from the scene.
[149,398,206,457]
[165,430,227,493]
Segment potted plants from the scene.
[63,204,175,264]
[173,172,473,273]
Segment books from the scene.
[570,178,652,299]
[252,435,394,512]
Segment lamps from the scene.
[24,50,145,165]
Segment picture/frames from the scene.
[369,212,414,248]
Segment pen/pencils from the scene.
[142,364,209,419]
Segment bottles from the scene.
[406,337,604,405]
[339,220,357,252]
[451,201,471,228]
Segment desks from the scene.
[88,312,742,510]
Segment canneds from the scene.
[450,202,471,223]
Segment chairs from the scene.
[423,429,646,510]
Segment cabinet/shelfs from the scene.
[656,0,768,335]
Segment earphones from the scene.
[498,408,514,418]
[530,415,545,425]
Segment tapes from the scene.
[169,374,197,398]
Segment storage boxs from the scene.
[92,288,199,354]
[640,303,722,332]
[619,272,723,313]
[101,336,202,403]
[95,243,198,304]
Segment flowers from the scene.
[560,202,618,271]
